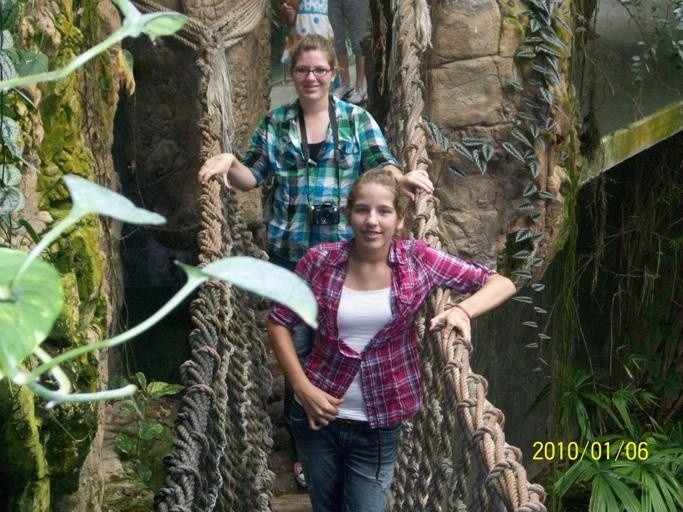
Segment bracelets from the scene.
[453,305,472,321]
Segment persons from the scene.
[267,169,518,512]
[198,33,435,488]
[280,0,373,104]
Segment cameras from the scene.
[311,203,339,225]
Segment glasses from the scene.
[293,66,334,78]
